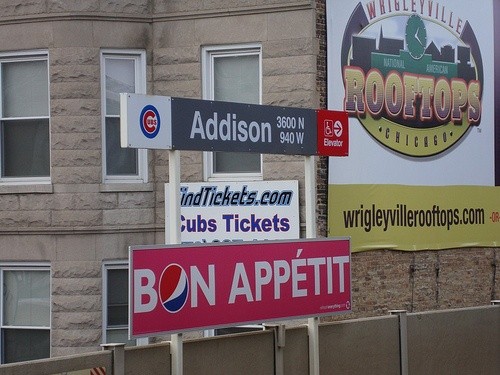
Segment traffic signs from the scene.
[317,109,349,157]
